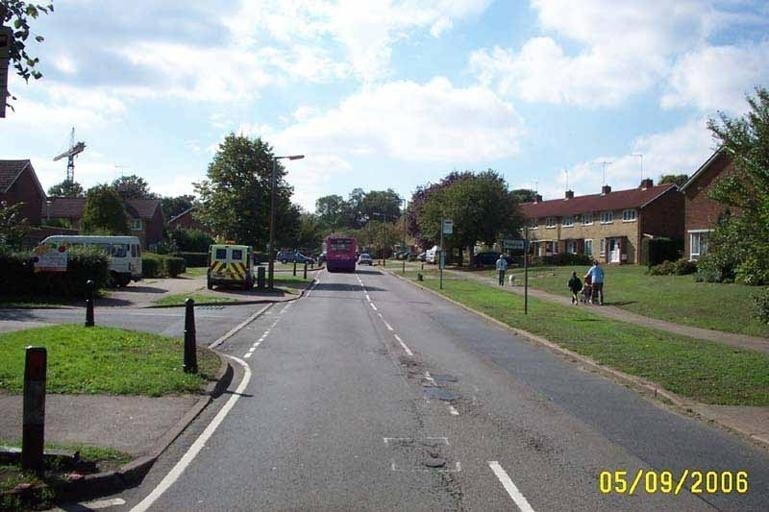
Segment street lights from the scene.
[373,212,387,267]
[267,155,304,289]
[386,197,407,273]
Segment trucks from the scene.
[325,235,357,272]
[207,241,256,291]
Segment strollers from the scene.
[578,275,593,304]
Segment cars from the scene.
[474,251,513,268]
[394,245,442,264]
[357,253,373,265]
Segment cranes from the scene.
[50,126,87,181]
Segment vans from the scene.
[276,249,316,265]
[31,233,143,288]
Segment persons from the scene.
[584,259,605,306]
[566,270,582,305]
[495,254,509,286]
